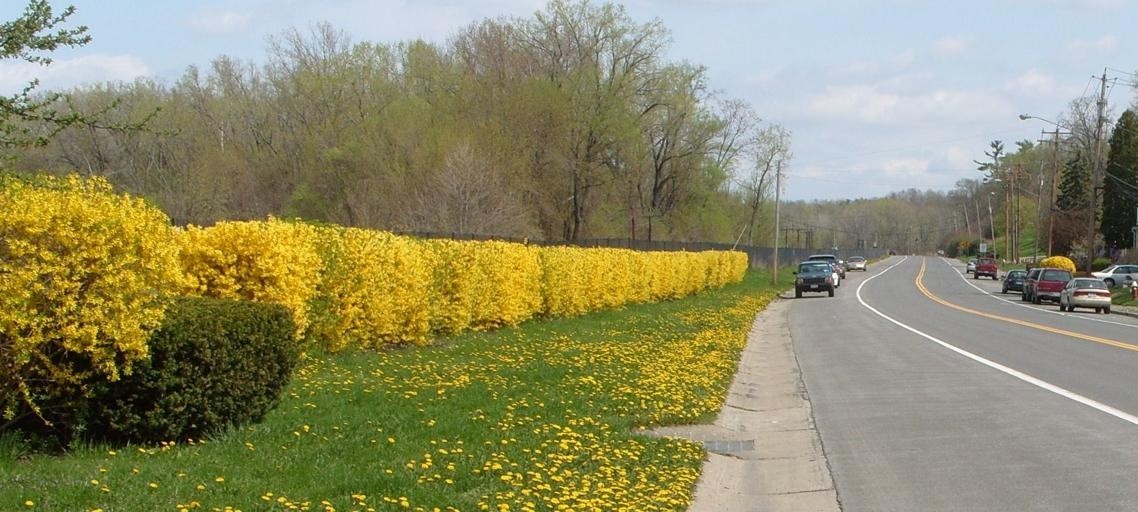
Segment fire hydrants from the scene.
[1130,280,1138,301]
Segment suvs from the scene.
[974,258,997,280]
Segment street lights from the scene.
[988,191,996,259]
[1019,114,1104,268]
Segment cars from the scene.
[1090,263,1138,287]
[793,255,867,297]
[1001,268,1112,315]
[967,259,978,273]
